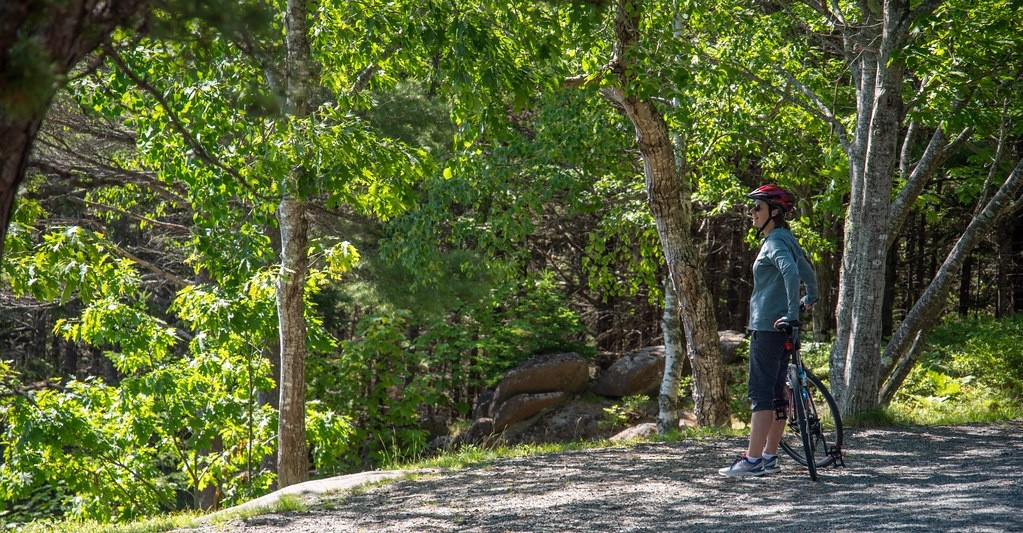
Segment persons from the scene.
[717,184,819,476]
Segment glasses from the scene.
[748,201,768,212]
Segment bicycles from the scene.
[744,295,847,482]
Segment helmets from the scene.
[746,185,795,213]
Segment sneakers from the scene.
[718,453,766,476]
[760,455,781,473]
